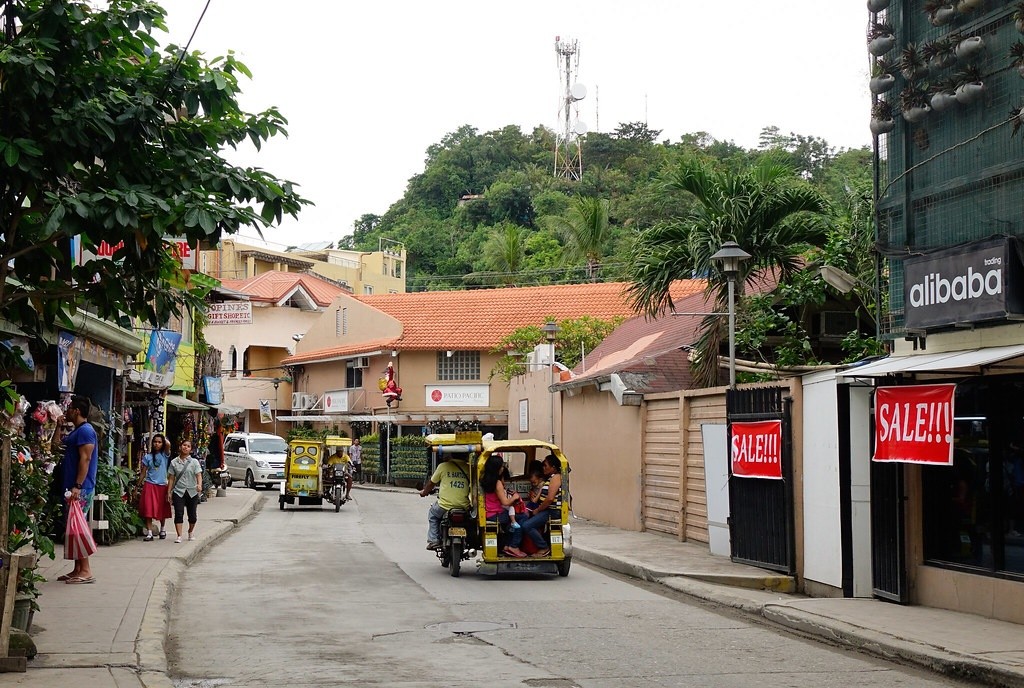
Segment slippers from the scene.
[502,550,508,553]
[506,547,527,558]
[58,574,71,581]
[531,549,550,557]
[66,576,95,584]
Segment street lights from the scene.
[710,240,751,394]
[271,377,282,435]
[539,321,563,444]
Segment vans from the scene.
[222,431,288,488]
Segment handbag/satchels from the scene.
[63,497,97,560]
[507,488,525,513]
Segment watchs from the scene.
[74,482,82,489]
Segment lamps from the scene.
[820,265,887,331]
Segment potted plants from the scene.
[8,437,61,630]
[920,0,985,28]
[1007,0,1024,141]
[900,32,984,120]
[866,0,895,136]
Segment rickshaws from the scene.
[416,430,574,577]
[279,434,353,513]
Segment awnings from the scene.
[164,393,245,415]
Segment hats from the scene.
[336,446,343,451]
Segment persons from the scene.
[324,437,365,500]
[53,396,100,584]
[420,452,562,559]
[136,434,203,543]
[228,370,254,378]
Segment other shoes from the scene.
[359,480,365,484]
[160,531,166,539]
[144,534,153,541]
[346,495,352,500]
[427,542,442,550]
[175,536,182,543]
[188,532,194,541]
[511,522,520,529]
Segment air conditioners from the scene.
[301,395,311,409]
[292,392,306,408]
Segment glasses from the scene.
[67,405,77,409]
